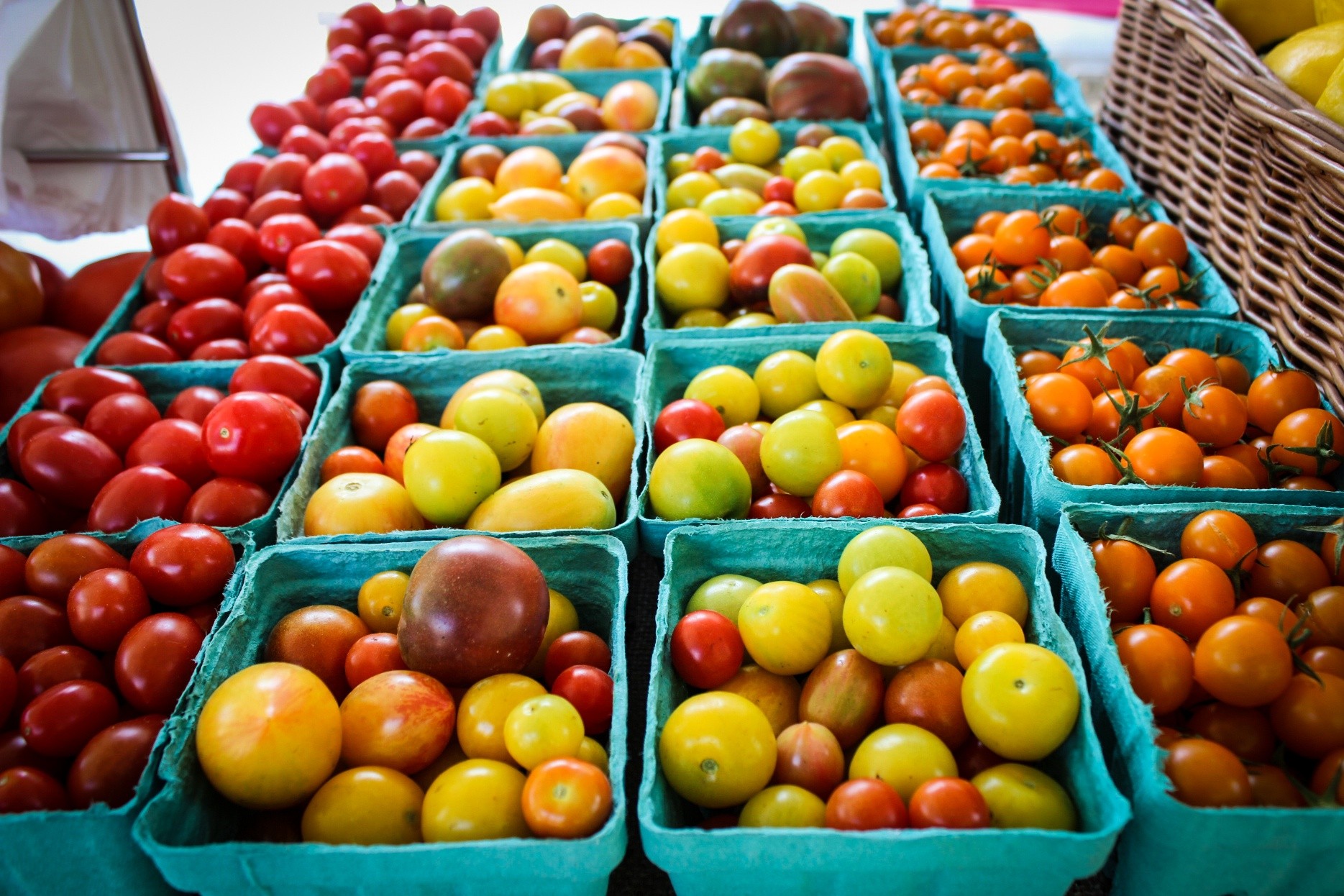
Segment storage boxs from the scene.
[2,0,1343,896]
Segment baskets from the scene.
[1098,0,1344,425]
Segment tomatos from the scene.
[865,0,1344,813]
[0,0,499,815]
[193,1,1082,845]
